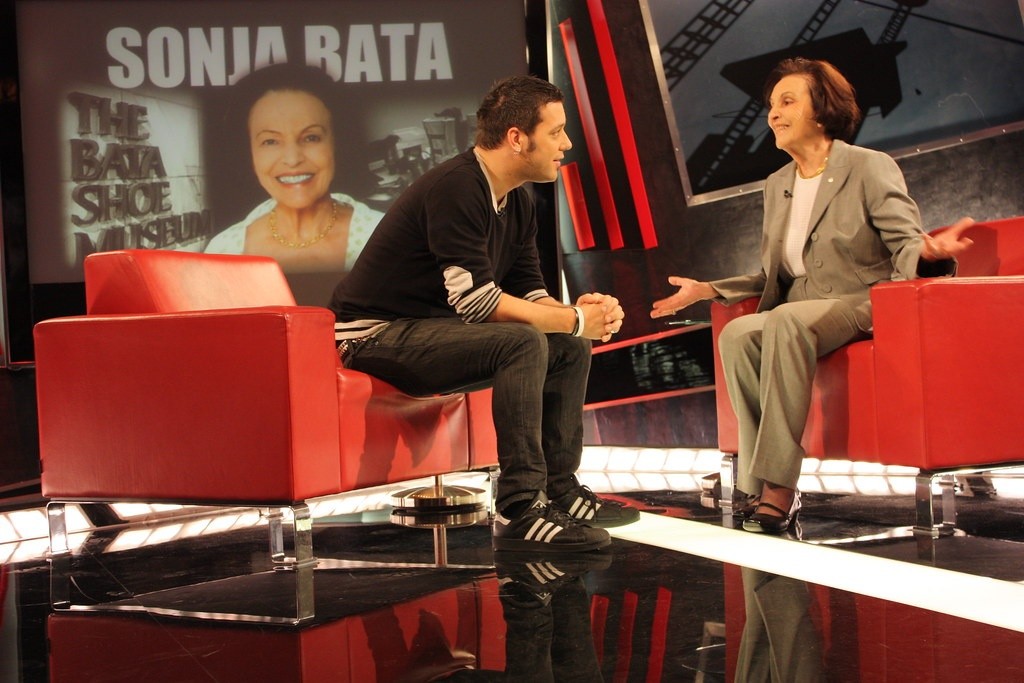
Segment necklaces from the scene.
[270,201,336,247]
[797,157,828,179]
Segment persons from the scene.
[203,64,385,272]
[732,519,826,683]
[328,75,640,552]
[435,551,613,683]
[650,58,974,533]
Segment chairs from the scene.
[23,522,507,683]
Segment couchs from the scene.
[700,214,1024,539]
[31,243,498,568]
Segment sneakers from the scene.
[492,491,611,553]
[546,485,640,528]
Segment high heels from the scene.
[743,494,802,532]
[732,488,801,520]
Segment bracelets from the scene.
[569,307,584,337]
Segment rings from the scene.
[673,310,676,315]
